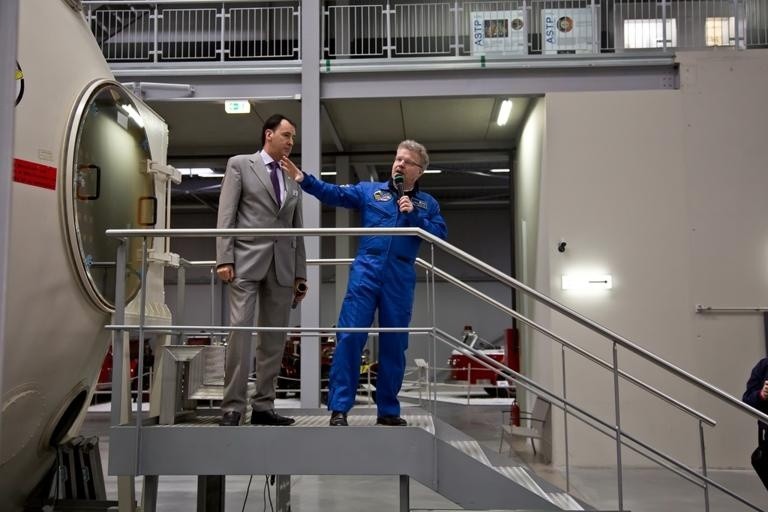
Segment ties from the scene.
[267,161,282,206]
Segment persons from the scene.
[277,139,448,426]
[745,358,768,488]
[215,115,308,426]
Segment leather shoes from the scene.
[219,412,242,425]
[251,410,295,425]
[329,410,348,426]
[378,415,407,426]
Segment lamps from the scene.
[497,100,513,126]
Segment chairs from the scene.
[499,395,551,455]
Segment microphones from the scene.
[394,174,407,213]
[291,284,306,309]
